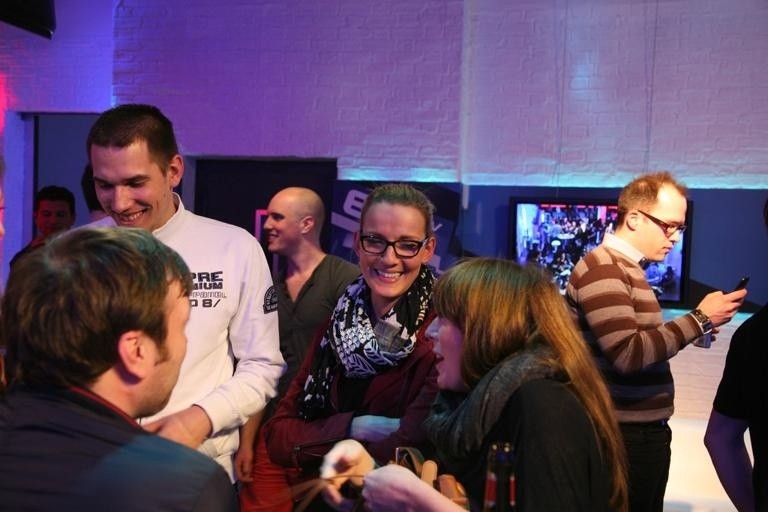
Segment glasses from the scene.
[357,226,430,258]
[638,207,687,236]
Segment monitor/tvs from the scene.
[508,196,693,310]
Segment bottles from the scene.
[479,442,522,511]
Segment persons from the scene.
[72,163,106,228]
[230,188,364,512]
[319,257,628,512]
[9,184,76,270]
[703,305,768,512]
[264,183,438,512]
[564,170,747,512]
[57,102,289,511]
[0,223,233,512]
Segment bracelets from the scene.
[691,309,712,333]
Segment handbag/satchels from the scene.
[239,411,302,512]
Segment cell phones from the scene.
[734,275,750,290]
